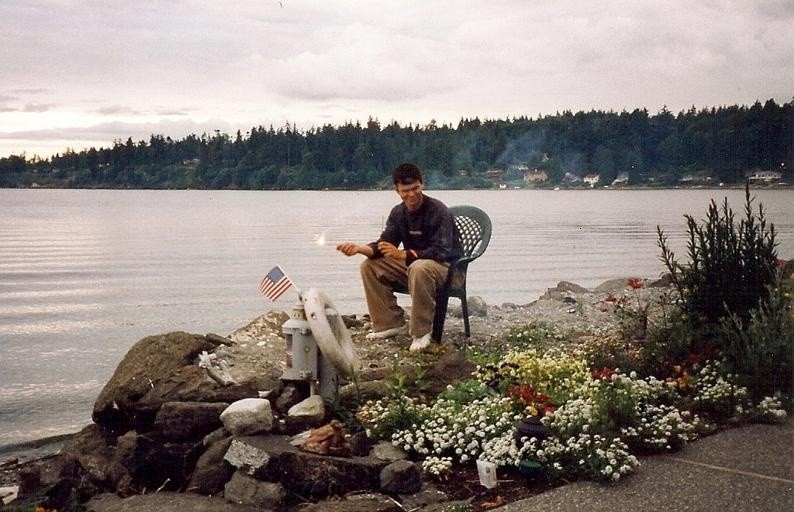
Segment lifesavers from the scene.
[304,286,359,378]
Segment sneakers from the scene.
[409,332,432,353]
[366,322,410,339]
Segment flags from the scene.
[257,265,294,303]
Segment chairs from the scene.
[393,206,493,342]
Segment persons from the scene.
[336,162,465,352]
